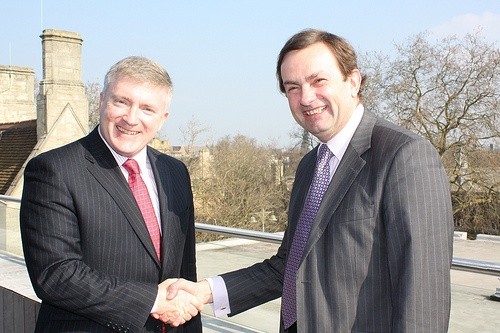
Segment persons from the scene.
[19,55,202,333]
[153,28,454,333]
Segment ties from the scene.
[122,159,169,333]
[282,144,334,330]
